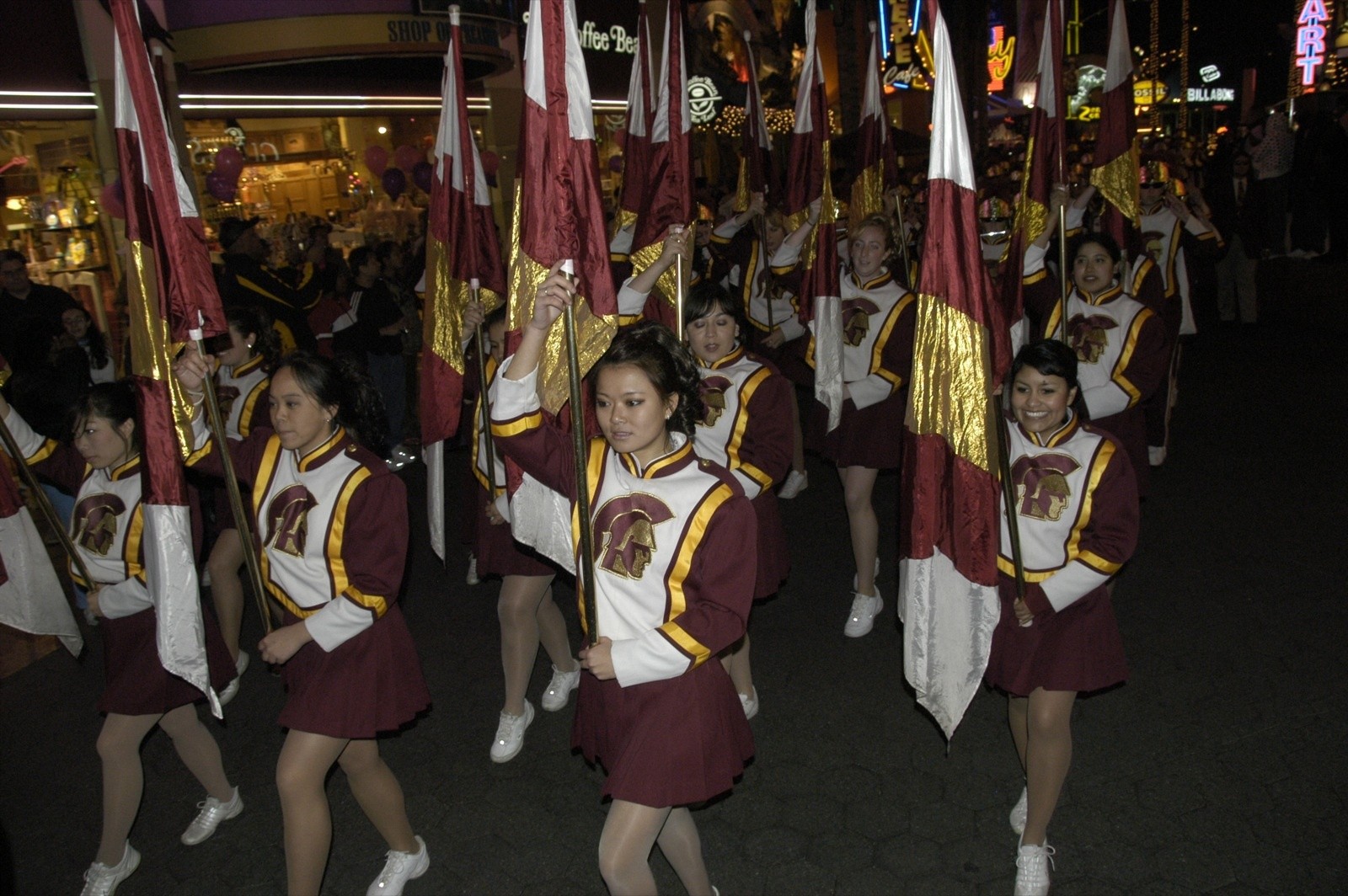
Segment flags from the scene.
[112,1,1143,743]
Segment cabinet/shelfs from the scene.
[0,124,362,277]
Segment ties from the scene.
[1237,181,1244,215]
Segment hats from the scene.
[218,215,260,250]
[978,196,1013,220]
[1138,160,1170,185]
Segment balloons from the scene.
[206,171,235,204]
[479,149,499,173]
[215,148,244,185]
[364,145,388,175]
[380,167,406,202]
[411,161,433,194]
[394,145,418,171]
[99,184,126,221]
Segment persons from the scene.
[0,111,1295,896]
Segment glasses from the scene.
[980,218,1007,223]
[1139,181,1168,189]
[64,315,86,325]
[1233,161,1249,166]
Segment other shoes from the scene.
[1219,321,1234,331]
[1242,322,1258,335]
[711,885,719,896]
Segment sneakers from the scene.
[542,658,581,712]
[366,837,430,896]
[219,650,249,706]
[80,839,141,896]
[854,557,879,591]
[1013,831,1056,896]
[778,469,808,499]
[844,583,883,637]
[181,785,244,845]
[392,443,417,463]
[384,459,405,472]
[490,698,535,763]
[1010,787,1027,835]
[1148,445,1166,465]
[737,685,759,720]
